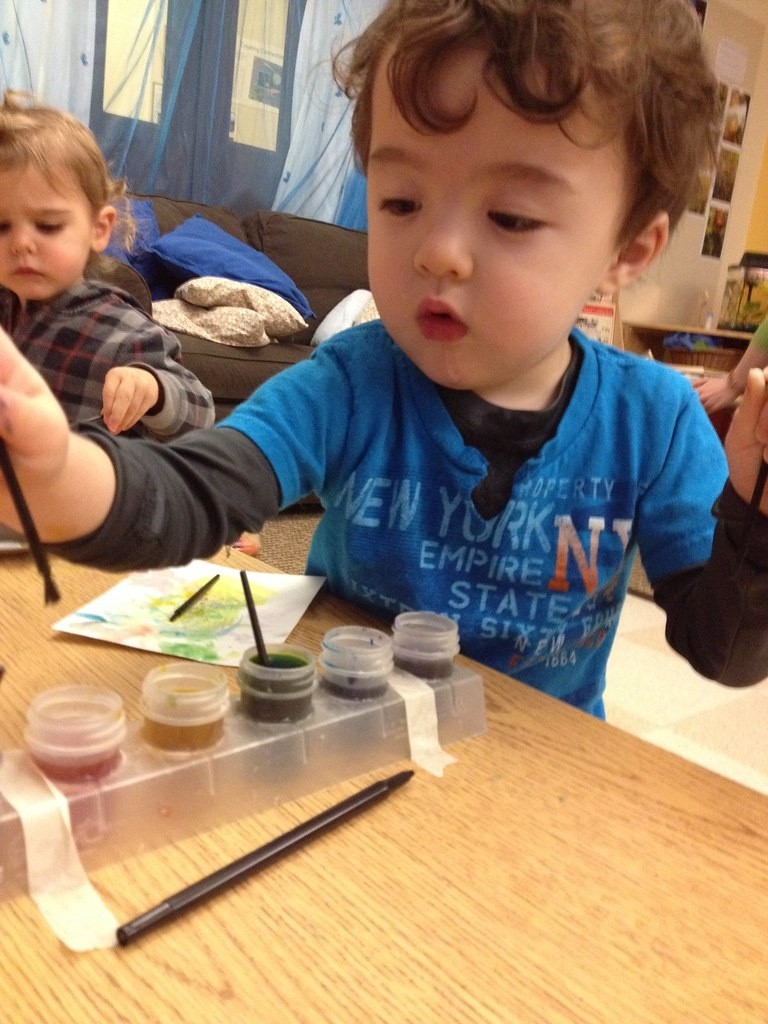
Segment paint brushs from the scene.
[0,435,60,604]
[170,575,221,623]
[116,769,416,948]
[239,569,270,665]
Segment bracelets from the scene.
[728,369,745,395]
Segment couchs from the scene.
[102,194,368,429]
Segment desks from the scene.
[625,319,756,341]
[0,520,768,1022]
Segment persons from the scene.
[0,91,215,440]
[0,1,768,720]
[693,314,768,414]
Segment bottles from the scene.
[22,612,458,780]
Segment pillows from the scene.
[143,214,321,325]
[104,197,162,278]
[170,274,310,335]
[149,298,278,347]
